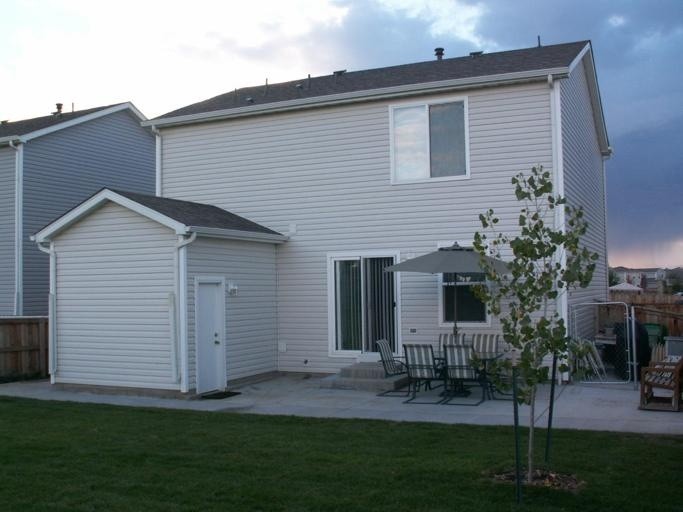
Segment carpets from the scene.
[202,391,241,399]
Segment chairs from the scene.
[638,355,683,412]
[376,333,548,406]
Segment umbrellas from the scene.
[381,239,520,346]
